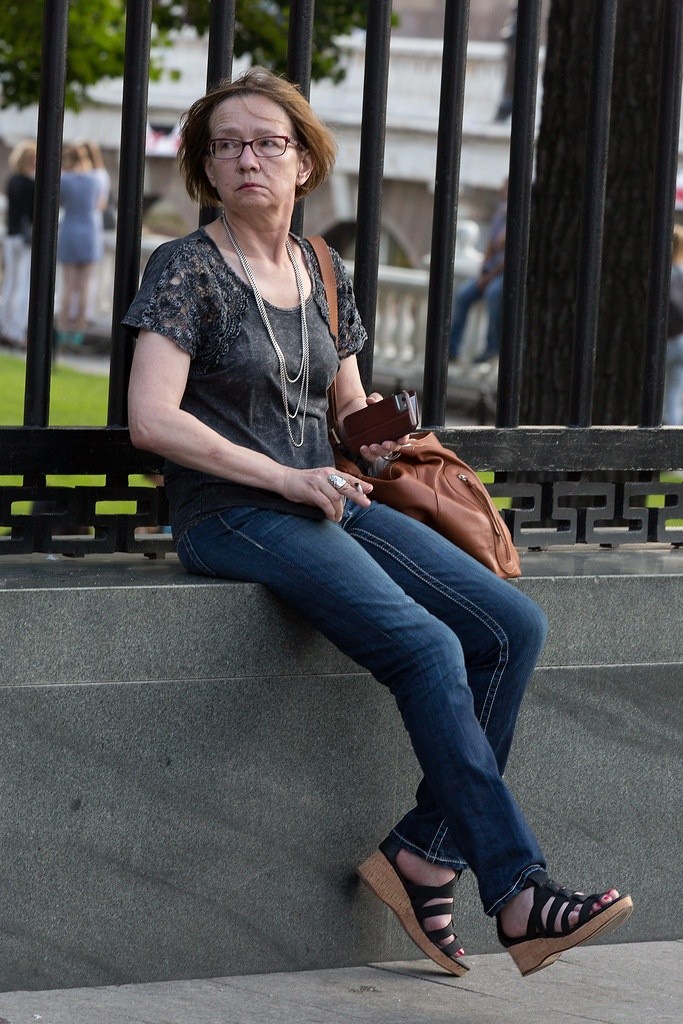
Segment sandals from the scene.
[356,836,471,977]
[496,870,633,977]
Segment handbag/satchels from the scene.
[334,431,522,580]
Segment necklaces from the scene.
[221,209,310,447]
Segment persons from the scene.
[448,176,509,366]
[661,225,683,431]
[0,138,37,352]
[56,141,110,349]
[120,67,635,975]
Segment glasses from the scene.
[207,134,306,158]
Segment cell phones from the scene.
[343,388,420,454]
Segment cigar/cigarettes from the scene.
[353,483,367,499]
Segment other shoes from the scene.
[0,336,27,350]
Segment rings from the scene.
[326,472,346,488]
